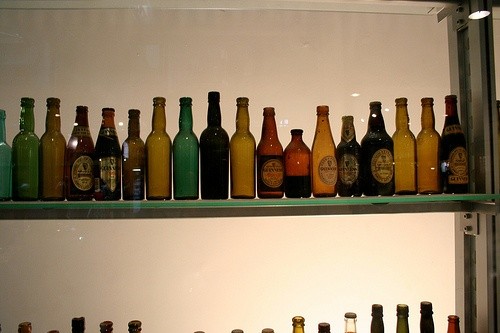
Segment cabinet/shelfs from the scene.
[0,0,500,333]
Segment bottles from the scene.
[283,129,313,198]
[172,97,200,199]
[199,91,230,199]
[94,108,122,202]
[39,97,68,201]
[361,101,396,196]
[310,105,338,197]
[441,95,470,194]
[145,96,173,199]
[256,107,285,198]
[12,97,41,202]
[337,116,363,197]
[415,97,441,194]
[121,109,147,201]
[66,105,95,201]
[18,315,142,333]
[230,97,256,199]
[392,97,417,195]
[193,301,460,333]
[0,109,13,201]
